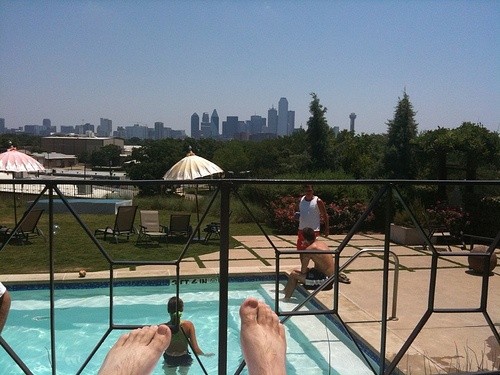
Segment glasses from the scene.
[304,190,312,193]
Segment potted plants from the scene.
[392,203,432,244]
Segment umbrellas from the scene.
[0,142,46,232]
[162,152,223,244]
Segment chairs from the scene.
[93,205,139,244]
[162,214,194,242]
[0,209,45,246]
[202,211,234,244]
[136,209,169,246]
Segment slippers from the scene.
[338,274,351,283]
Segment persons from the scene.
[159,296,213,374]
[269,230,335,302]
[0,281,12,334]
[294,184,328,272]
[96,299,286,375]
